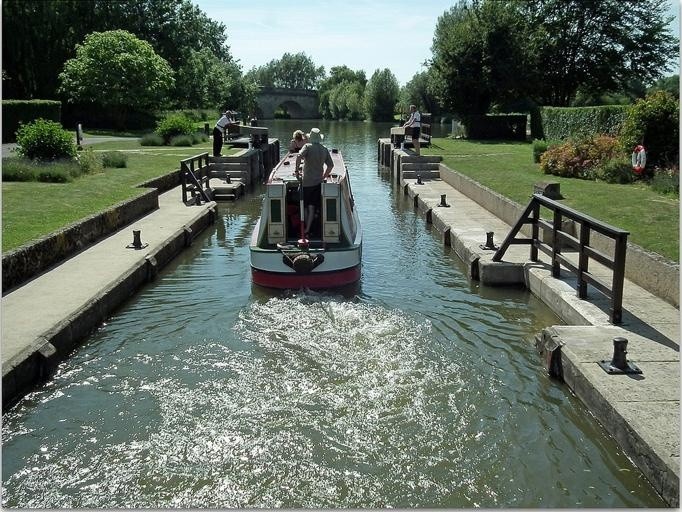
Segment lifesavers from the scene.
[631,145,646,174]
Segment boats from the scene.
[247,146,365,293]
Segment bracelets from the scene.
[295,170,299,173]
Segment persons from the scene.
[294,127,335,239]
[212,110,241,157]
[291,129,307,152]
[402,105,421,155]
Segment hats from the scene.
[306,127,324,143]
